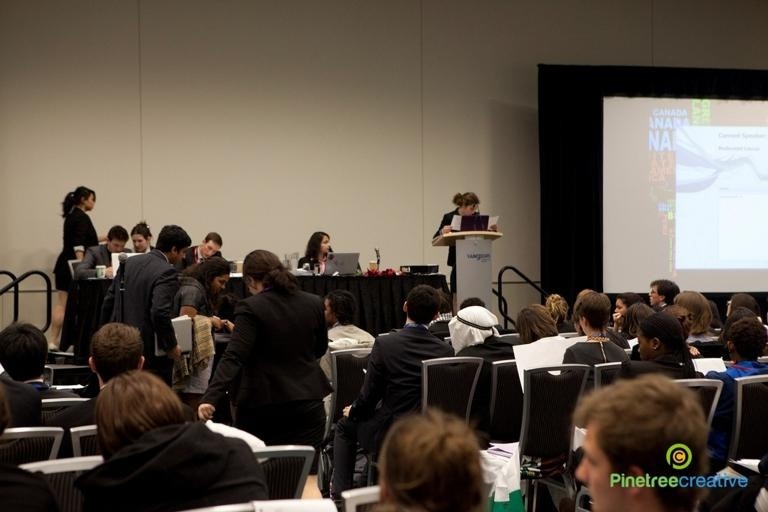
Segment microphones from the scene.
[472,203,480,215]
[118,253,127,282]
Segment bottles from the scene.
[283,254,298,270]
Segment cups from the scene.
[236,261,243,273]
[369,261,378,270]
[302,263,310,271]
[96,265,106,279]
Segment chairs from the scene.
[0,324,768,509]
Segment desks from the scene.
[66,269,449,362]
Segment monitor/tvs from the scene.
[460,216,490,231]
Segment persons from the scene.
[433,190,497,316]
[131,220,154,254]
[0,225,768,511]
[48,186,107,351]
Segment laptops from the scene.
[111,252,143,280]
[325,252,360,275]
